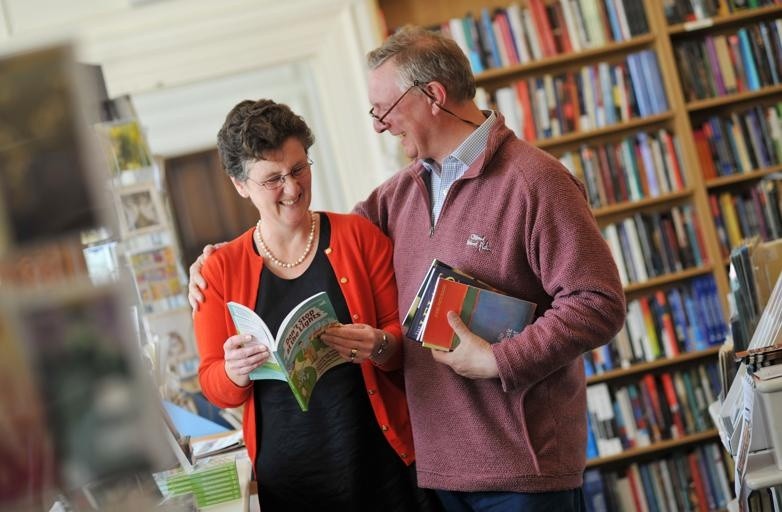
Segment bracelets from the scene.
[369,330,391,364]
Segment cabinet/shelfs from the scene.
[2,49,249,512]
[378,0,782,512]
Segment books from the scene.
[430,1,782,510]
[226,289,354,414]
[0,103,247,508]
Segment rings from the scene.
[350,348,359,360]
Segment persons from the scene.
[184,21,630,510]
[190,96,428,510]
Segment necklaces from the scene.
[255,210,315,268]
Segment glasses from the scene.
[245,156,315,191]
